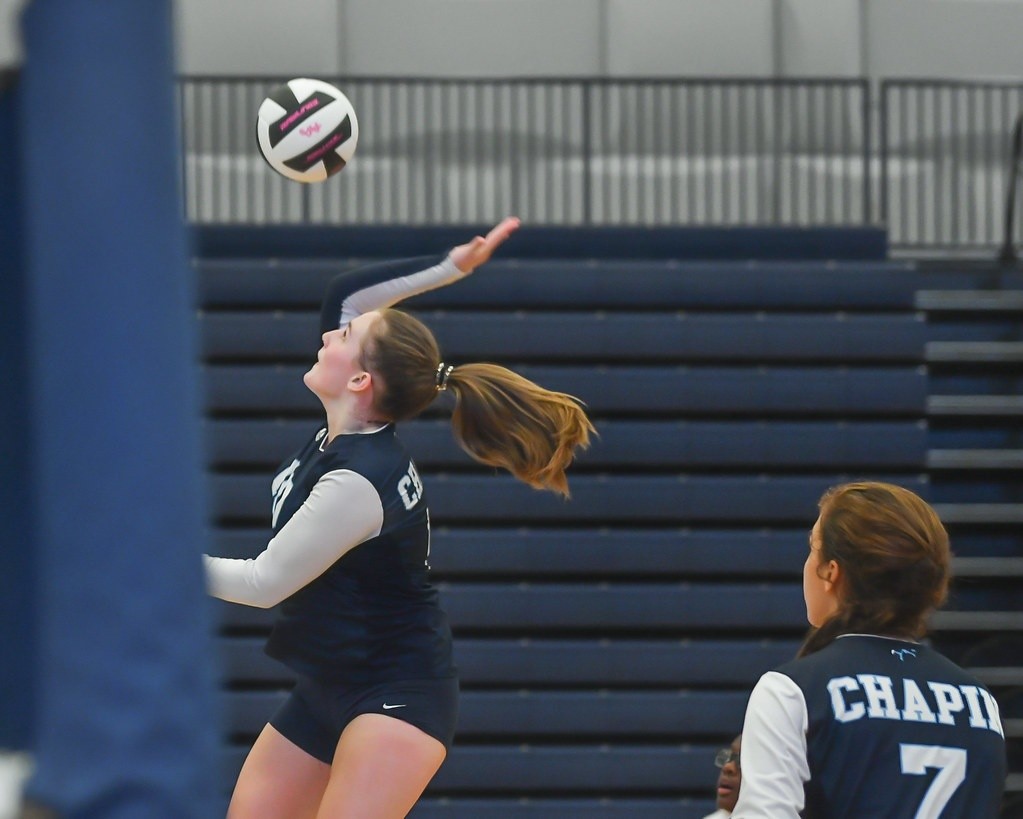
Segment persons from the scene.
[730,481,1006,819]
[703,735,743,819]
[202,216,600,819]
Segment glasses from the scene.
[714,748,741,770]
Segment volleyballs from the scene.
[256,78,360,184]
